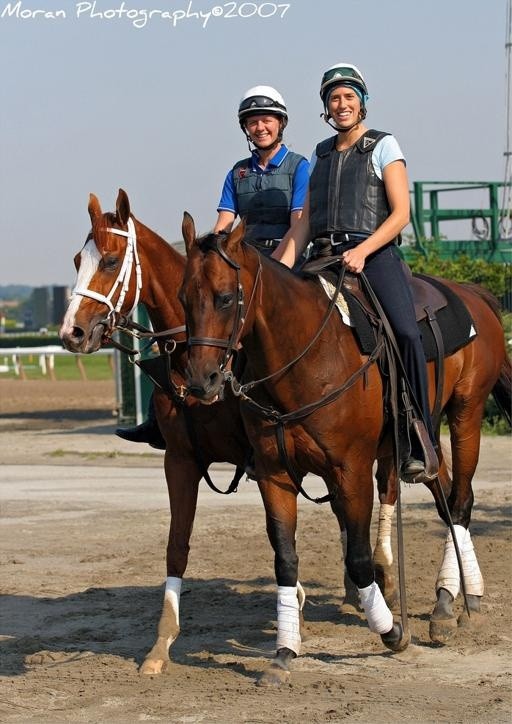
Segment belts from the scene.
[259,240,280,248]
[324,233,367,247]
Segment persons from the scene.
[113,84,312,449]
[275,62,426,477]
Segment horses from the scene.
[176,209,511,689]
[56,187,400,675]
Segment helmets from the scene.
[237,86,288,122]
[320,63,368,100]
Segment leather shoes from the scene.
[114,420,168,448]
[402,456,425,474]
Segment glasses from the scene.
[322,67,366,90]
[239,95,287,111]
